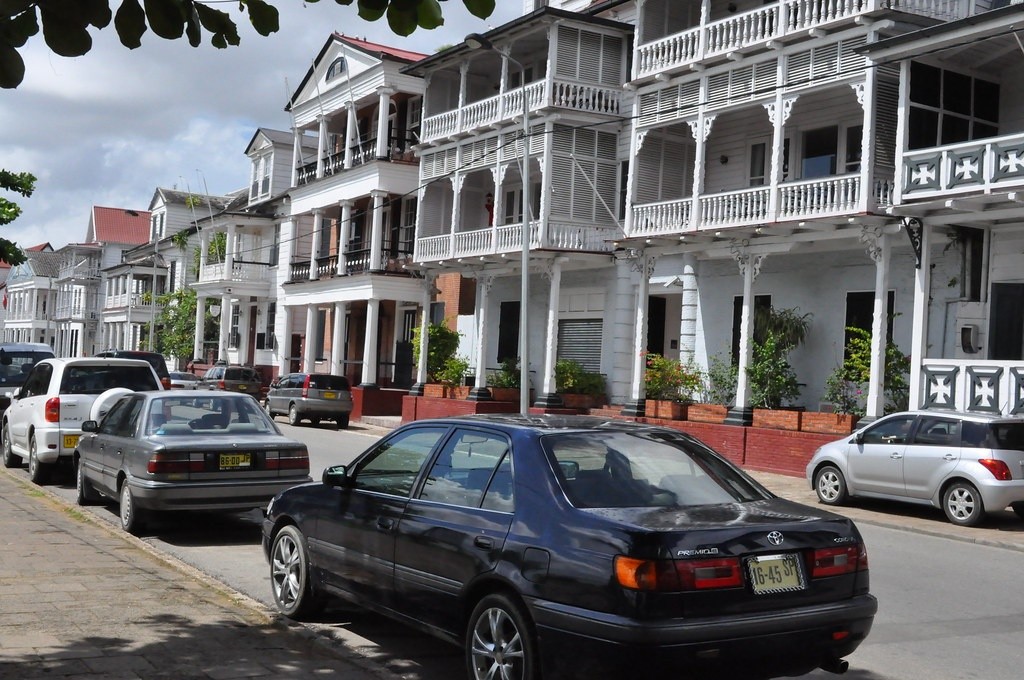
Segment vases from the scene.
[802,411,860,436]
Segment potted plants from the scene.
[744,329,802,431]
[409,314,464,398]
[553,357,610,410]
[435,357,474,400]
[688,354,739,423]
[484,356,535,402]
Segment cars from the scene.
[264,372,353,429]
[74,390,314,534]
[262,414,878,680]
[0,342,263,485]
[806,410,1024,526]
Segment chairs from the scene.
[574,468,613,503]
[467,468,510,496]
[202,414,229,429]
[930,428,947,444]
[152,414,167,427]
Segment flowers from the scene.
[640,350,702,405]
[822,367,867,420]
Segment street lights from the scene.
[464,33,529,413]
